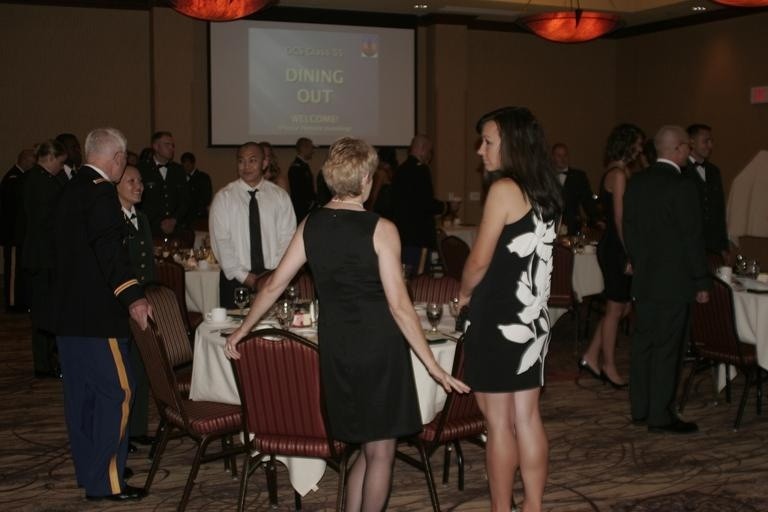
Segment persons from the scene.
[640,142,657,170]
[363,136,463,272]
[548,143,602,239]
[208,141,297,309]
[258,141,289,191]
[224,136,471,509]
[2,150,35,313]
[115,166,156,455]
[140,147,155,160]
[457,107,568,508]
[56,133,83,188]
[680,123,728,373]
[181,152,213,218]
[622,126,715,435]
[133,131,193,240]
[316,169,335,208]
[288,138,316,227]
[35,127,155,501]
[579,123,647,389]
[21,139,69,378]
[126,151,138,168]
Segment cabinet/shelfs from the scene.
[286,286,299,306]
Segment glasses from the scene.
[167,0,267,22]
[522,0,619,43]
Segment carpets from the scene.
[70,170,76,178]
[244,190,265,275]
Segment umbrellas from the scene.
[647,419,699,434]
[122,467,134,479]
[85,483,147,500]
[633,416,649,425]
[33,367,62,379]
[128,435,157,445]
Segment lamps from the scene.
[149,260,202,335]
[127,314,242,512]
[548,243,580,357]
[145,287,202,460]
[256,269,315,303]
[150,229,195,255]
[394,332,489,511]
[676,275,767,428]
[408,270,461,303]
[226,327,346,512]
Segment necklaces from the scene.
[331,199,363,208]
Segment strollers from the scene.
[157,164,168,170]
[694,161,706,170]
[129,213,139,219]
[555,171,569,177]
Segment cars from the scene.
[206,307,226,323]
[720,266,732,285]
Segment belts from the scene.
[570,235,579,253]
[580,232,587,252]
[449,299,458,334]
[275,300,294,331]
[233,288,251,322]
[427,301,443,336]
[746,256,760,283]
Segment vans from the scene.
[577,357,603,380]
[599,366,628,388]
